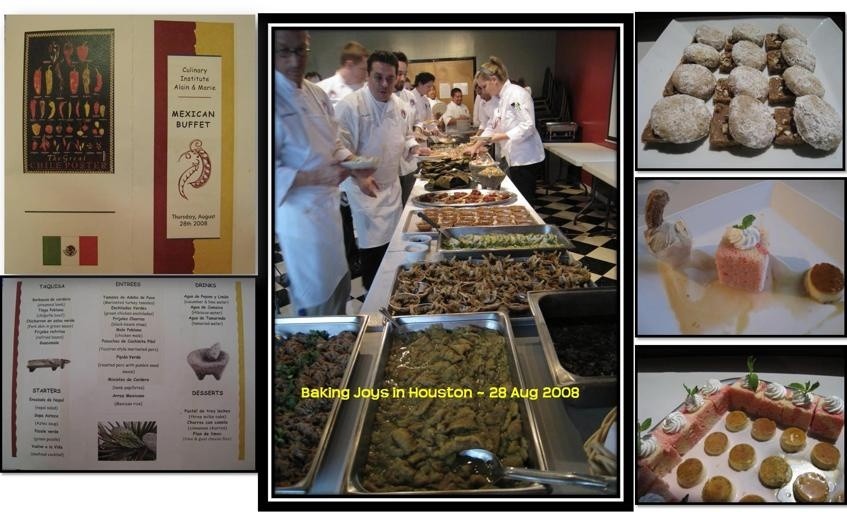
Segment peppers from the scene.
[28,41,106,151]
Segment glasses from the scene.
[276,46,313,57]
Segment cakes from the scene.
[804,262,844,303]
[637,355,845,503]
[643,188,692,268]
[714,214,770,293]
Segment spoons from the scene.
[455,448,611,492]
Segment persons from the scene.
[268,26,548,316]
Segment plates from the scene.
[636,15,842,167]
[416,155,444,161]
[410,190,516,208]
[338,160,376,170]
[633,180,846,345]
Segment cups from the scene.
[403,243,428,261]
[408,234,433,251]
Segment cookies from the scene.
[641,23,843,152]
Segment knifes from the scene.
[416,211,455,240]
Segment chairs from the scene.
[531,65,577,140]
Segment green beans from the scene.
[189,147,211,188]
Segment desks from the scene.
[580,162,618,238]
[541,141,620,226]
